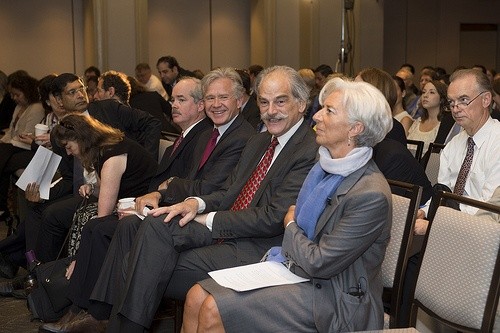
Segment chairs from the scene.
[345,139,500,333]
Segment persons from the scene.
[39,68,258,333]
[391,68,500,328]
[45,77,214,333]
[0,56,334,322]
[180,76,393,332]
[355,64,500,204]
[108,65,320,333]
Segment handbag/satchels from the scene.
[24,255,75,324]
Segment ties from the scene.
[171,131,183,157]
[198,128,220,171]
[228,137,279,210]
[453,137,475,196]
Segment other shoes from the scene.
[12,286,31,299]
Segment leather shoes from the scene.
[38,309,109,333]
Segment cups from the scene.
[35,124,49,145]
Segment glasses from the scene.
[445,90,487,111]
[58,88,87,97]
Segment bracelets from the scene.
[286,220,294,227]
[89,182,94,195]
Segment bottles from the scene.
[118,197,135,211]
